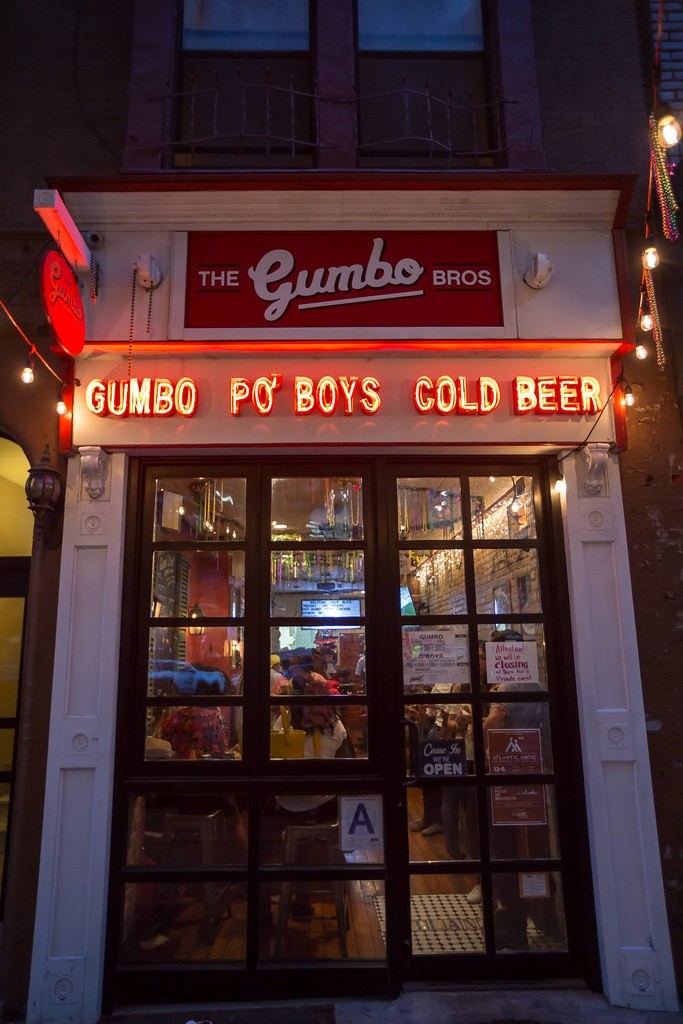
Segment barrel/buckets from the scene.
[270,705,306,759]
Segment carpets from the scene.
[371,891,564,956]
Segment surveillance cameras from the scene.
[85,230,104,250]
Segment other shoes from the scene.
[421,823,445,836]
[409,818,430,831]
[467,884,482,903]
[471,851,479,859]
[448,852,465,860]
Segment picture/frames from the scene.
[491,578,513,632]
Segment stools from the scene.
[151,809,235,933]
[274,821,353,958]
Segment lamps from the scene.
[185,602,207,636]
[24,443,65,550]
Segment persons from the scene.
[124,646,347,924]
[467,639,501,904]
[356,649,366,685]
[403,681,482,889]
[482,629,560,952]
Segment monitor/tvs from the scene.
[400,586,420,629]
[301,598,362,630]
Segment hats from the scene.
[270,654,280,668]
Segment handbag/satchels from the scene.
[237,704,306,758]
